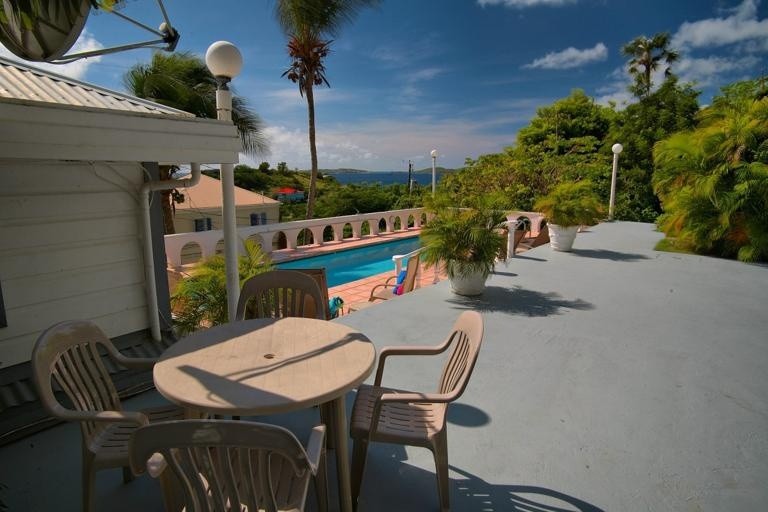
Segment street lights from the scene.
[428,147,439,204]
[606,142,625,222]
[205,33,253,324]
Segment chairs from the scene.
[235,270,328,322]
[30,319,209,512]
[348,310,484,512]
[127,418,329,511]
[366,254,420,303]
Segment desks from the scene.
[153,316,378,512]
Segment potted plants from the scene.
[533,179,595,252]
[417,195,506,297]
